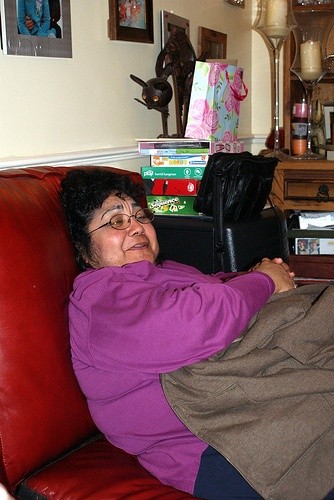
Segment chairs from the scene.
[216,162,291,271]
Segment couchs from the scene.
[0,166,212,500]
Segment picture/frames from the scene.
[0,0,73,58]
[197,27,228,62]
[107,0,155,45]
[160,10,190,50]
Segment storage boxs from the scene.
[138,141,215,215]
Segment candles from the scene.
[300,40,322,80]
[265,0,289,39]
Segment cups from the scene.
[291,102,308,156]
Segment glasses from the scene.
[88,208,155,234]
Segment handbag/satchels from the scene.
[185,61,248,142]
[193,151,279,224]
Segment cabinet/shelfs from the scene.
[264,0,334,288]
[154,215,217,276]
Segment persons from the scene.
[58,166,334,500]
[15,0,62,39]
